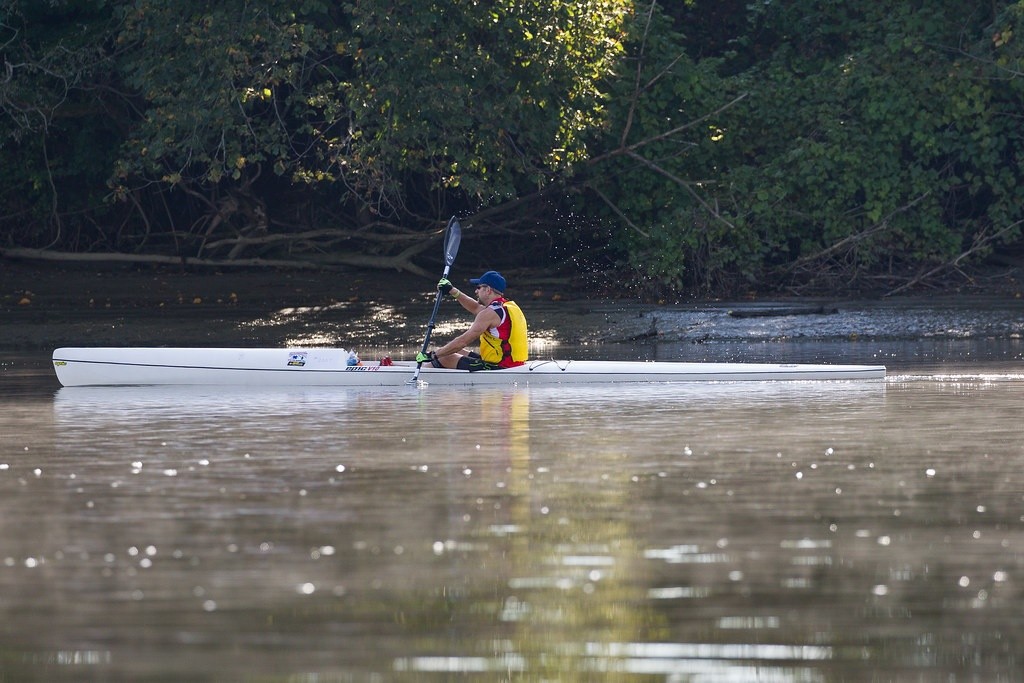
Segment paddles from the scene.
[405,215,462,386]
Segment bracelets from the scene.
[453,291,460,298]
[433,352,438,360]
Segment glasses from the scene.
[477,284,492,290]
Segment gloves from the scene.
[436,278,453,297]
[415,351,434,363]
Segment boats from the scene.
[53,344,890,401]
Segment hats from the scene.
[469,271,506,292]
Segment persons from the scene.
[416,271,528,371]
